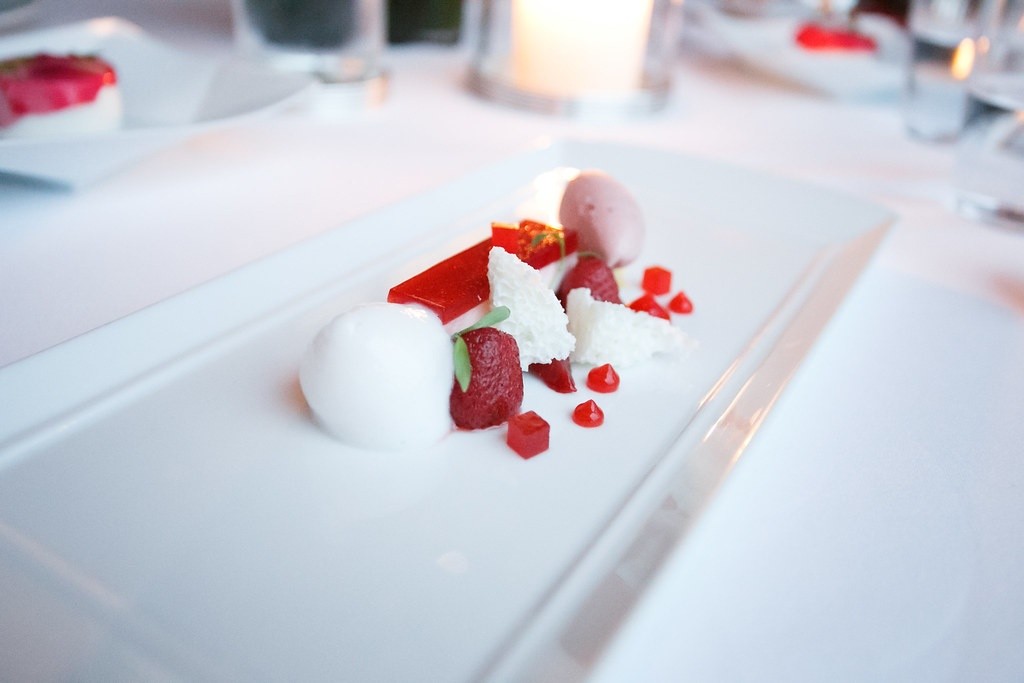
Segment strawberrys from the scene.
[553,256,623,313]
[448,327,523,430]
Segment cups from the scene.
[228,0,388,119]
[460,0,684,125]
[904,0,1024,231]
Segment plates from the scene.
[0,137,900,682]
[0,16,318,194]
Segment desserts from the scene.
[297,168,684,451]
[0,52,125,143]
